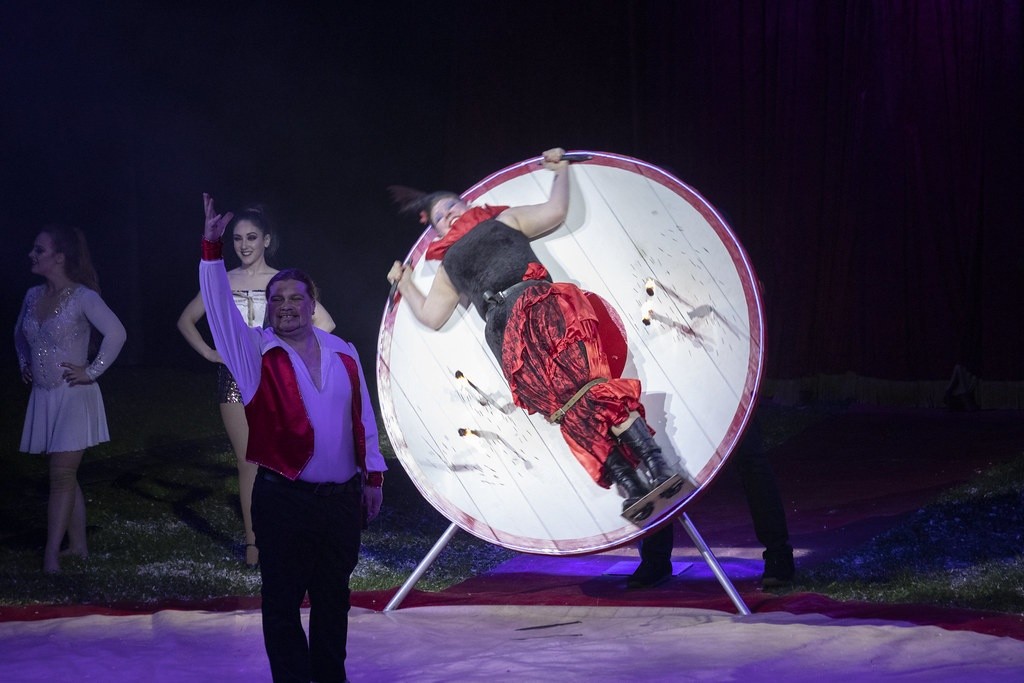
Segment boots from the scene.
[605,449,652,520]
[618,417,683,498]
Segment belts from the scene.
[483,279,549,319]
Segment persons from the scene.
[388,147,685,523]
[177,205,336,567]
[14,224,127,569]
[627,417,799,588]
[198,191,388,683]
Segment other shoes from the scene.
[245,543,259,571]
[761,556,793,586]
[627,557,673,591]
